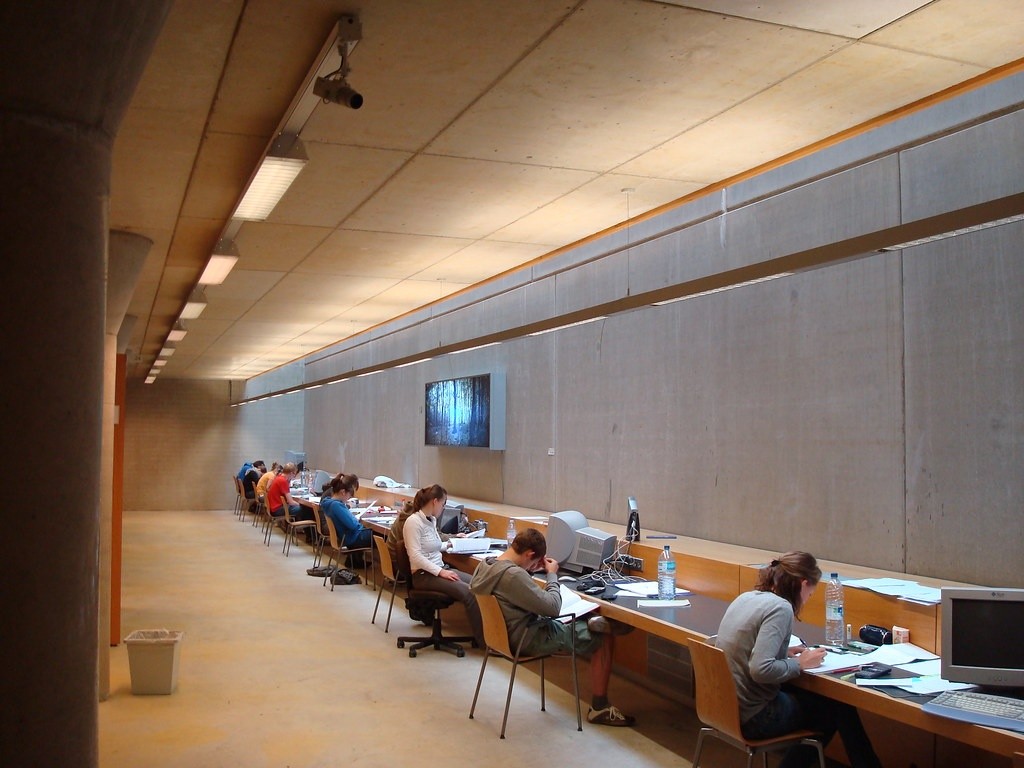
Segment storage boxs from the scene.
[892,625,910,644]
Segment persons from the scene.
[318,473,385,569]
[236,460,320,543]
[387,488,448,581]
[716,550,884,768]
[402,484,504,655]
[468,528,637,727]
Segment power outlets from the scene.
[632,557,644,572]
[483,522,488,532]
[474,520,479,529]
[618,553,633,570]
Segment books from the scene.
[787,647,873,675]
[538,584,600,624]
[446,537,508,554]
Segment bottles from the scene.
[657,546,676,600]
[825,572,844,642]
[506,520,516,550]
[308,472,313,484]
[301,472,306,486]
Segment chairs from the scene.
[394,539,479,658]
[260,484,267,533]
[371,535,407,633]
[323,512,372,592]
[280,495,321,558]
[311,503,330,568]
[263,494,299,547]
[252,481,265,528]
[686,636,826,768]
[233,475,242,516]
[238,478,263,522]
[469,593,583,740]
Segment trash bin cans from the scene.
[122,629,184,694]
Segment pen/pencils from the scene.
[830,668,857,673]
[840,673,855,680]
[799,638,810,650]
[646,536,677,539]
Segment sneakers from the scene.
[586,705,635,727]
[587,615,635,634]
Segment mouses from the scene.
[558,576,577,582]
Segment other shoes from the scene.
[345,561,374,569]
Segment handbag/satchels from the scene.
[306,563,336,576]
[331,569,363,584]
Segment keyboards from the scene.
[922,690,1024,733]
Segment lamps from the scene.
[166,325,189,342]
[153,356,167,367]
[144,374,157,385]
[149,366,162,375]
[230,133,309,223]
[159,343,176,357]
[178,289,209,320]
[196,239,241,287]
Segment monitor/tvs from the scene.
[546,510,617,575]
[940,586,1024,699]
[437,500,464,533]
[284,451,306,467]
[313,469,335,494]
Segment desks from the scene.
[292,469,1024,768]
